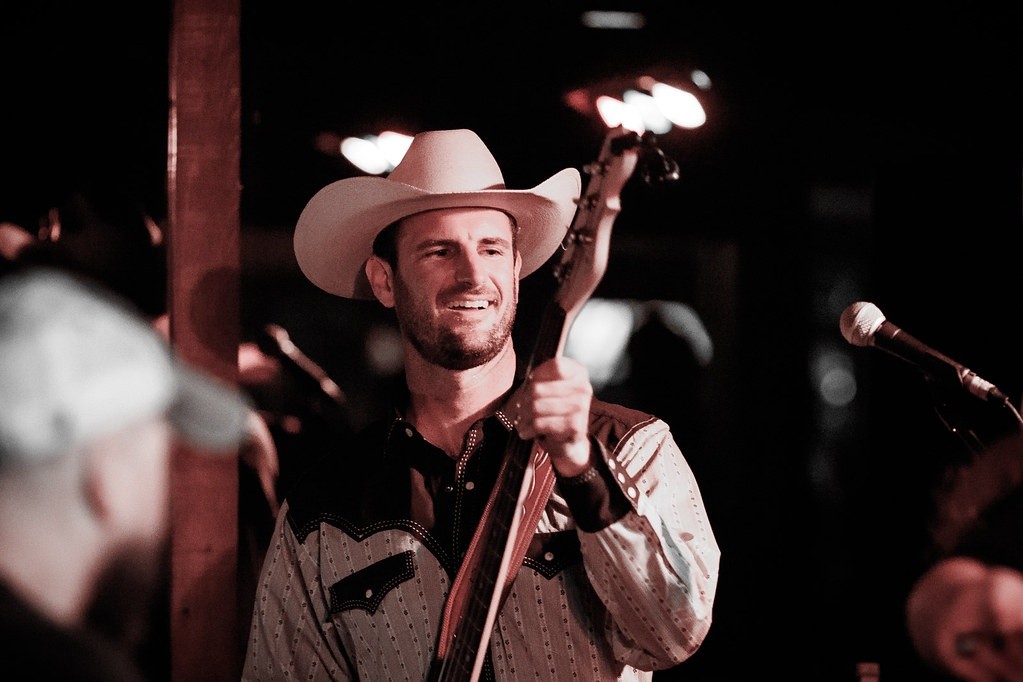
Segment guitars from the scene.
[431,124,681,682]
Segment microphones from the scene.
[257,324,343,404]
[838,302,1008,409]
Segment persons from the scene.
[241,130,722,682]
[0,240,278,682]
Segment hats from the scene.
[1,272,177,469]
[292,129,584,302]
